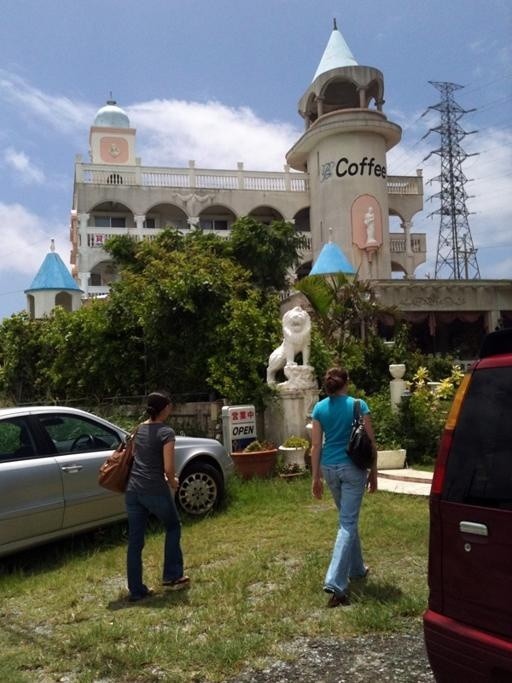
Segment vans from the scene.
[423,353,512,683]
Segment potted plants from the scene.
[279,463,305,484]
[368,391,407,470]
[231,440,278,483]
[278,433,308,469]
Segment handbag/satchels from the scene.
[347,417,377,471]
[96,441,135,492]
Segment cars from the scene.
[0,404,235,555]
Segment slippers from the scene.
[130,588,153,601]
[161,576,189,587]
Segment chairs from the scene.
[21,418,57,453]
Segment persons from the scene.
[126,392,189,602]
[365,207,374,243]
[311,367,377,598]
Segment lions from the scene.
[264,305,313,386]
